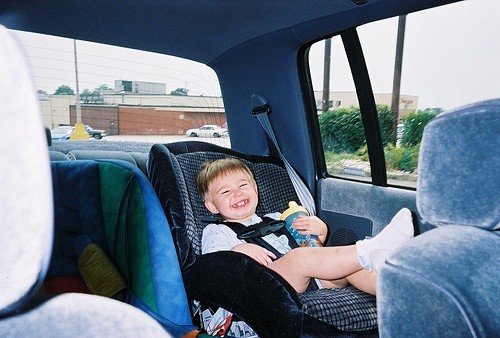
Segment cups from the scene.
[281,201,323,247]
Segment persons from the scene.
[197,157,414,295]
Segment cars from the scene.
[220,122,230,136]
[83,125,107,140]
[51,125,73,140]
[186,125,223,138]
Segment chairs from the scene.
[148,141,378,338]
[0,24,173,337]
[12,156,222,338]
[376,97,500,338]
